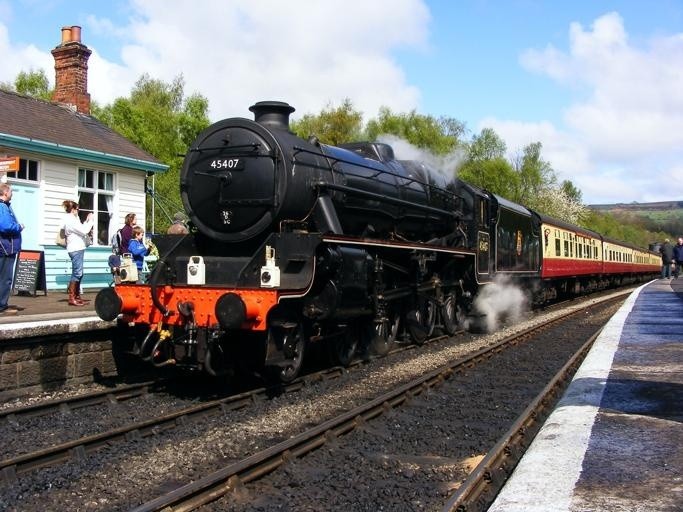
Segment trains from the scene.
[94,101,677,389]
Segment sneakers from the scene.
[0,308,18,315]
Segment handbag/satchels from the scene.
[56,229,67,248]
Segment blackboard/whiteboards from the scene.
[11,251,41,290]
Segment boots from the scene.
[68,281,89,306]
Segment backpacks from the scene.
[111,229,123,254]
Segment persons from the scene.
[126,226,153,285]
[109,248,121,277]
[167,212,189,234]
[61,201,94,306]
[545,229,550,246]
[673,238,683,279]
[0,183,24,316]
[659,238,673,280]
[119,214,136,256]
